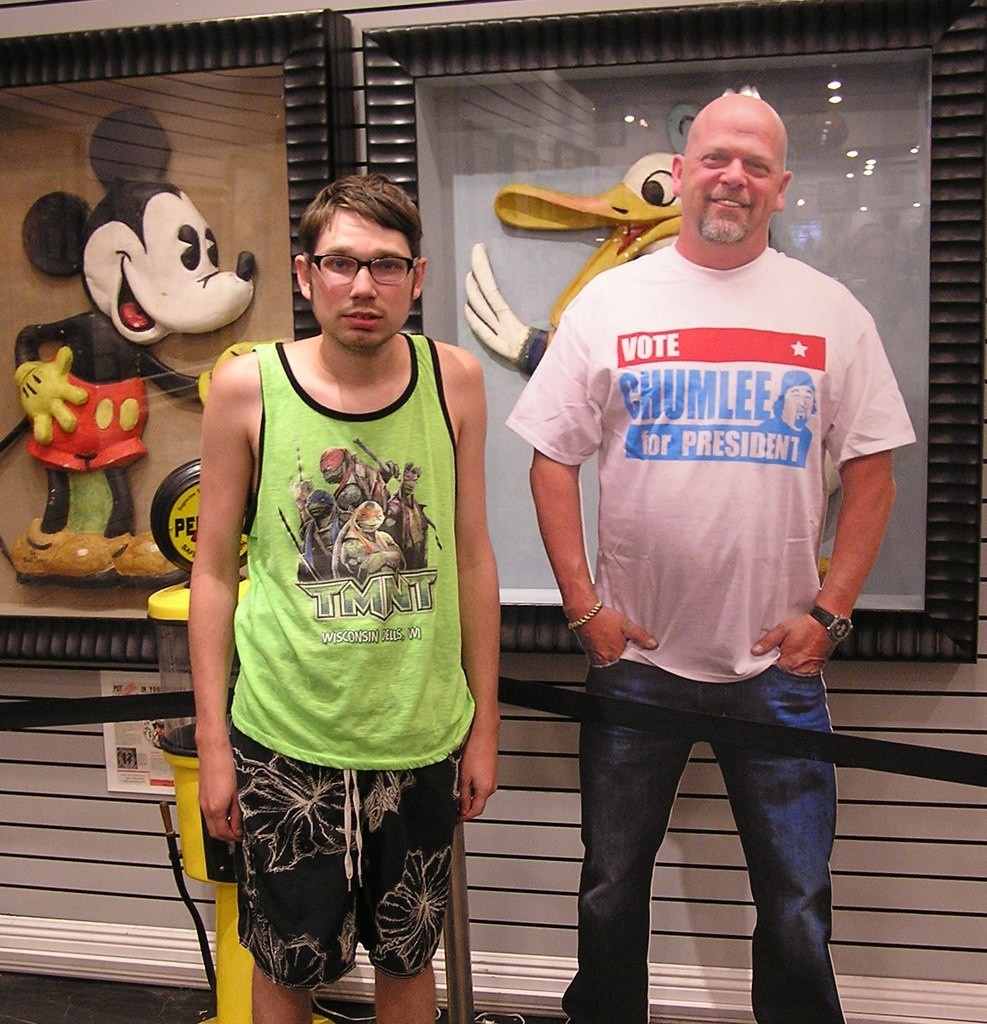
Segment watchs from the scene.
[810,606,852,641]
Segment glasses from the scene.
[304,255,420,286]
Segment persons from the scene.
[506,96,915,1024]
[188,180,500,1024]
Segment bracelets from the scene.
[568,600,603,629]
